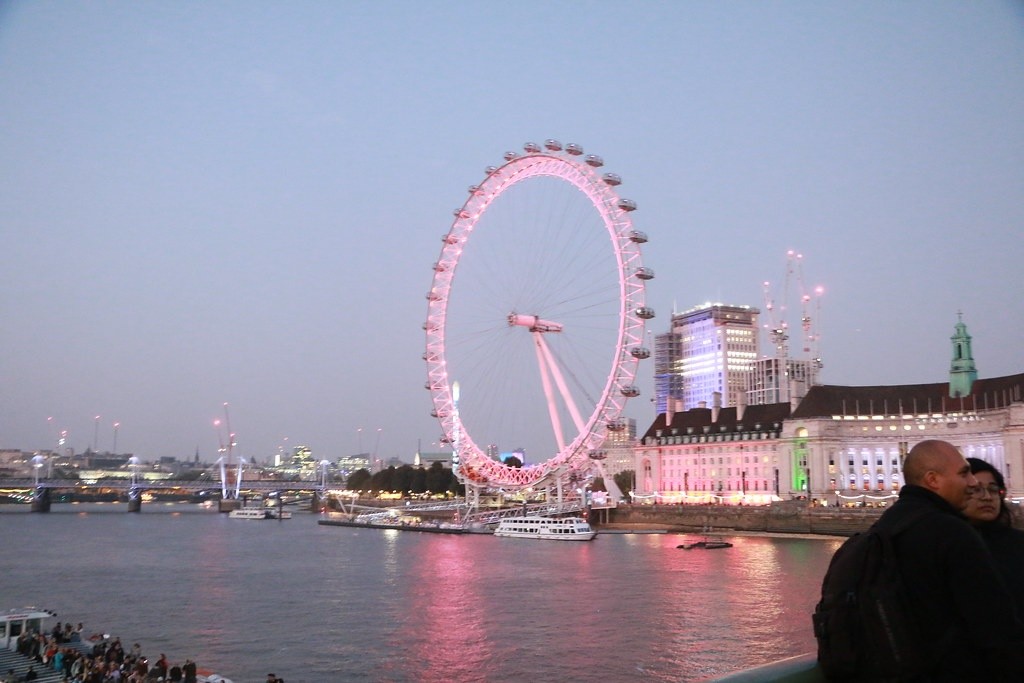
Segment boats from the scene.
[494,516,599,541]
[228,509,266,519]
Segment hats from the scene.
[65,623,73,629]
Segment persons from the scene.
[812,440,1024,683]
[4,621,196,683]
[266,673,284,683]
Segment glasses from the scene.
[971,486,1007,499]
